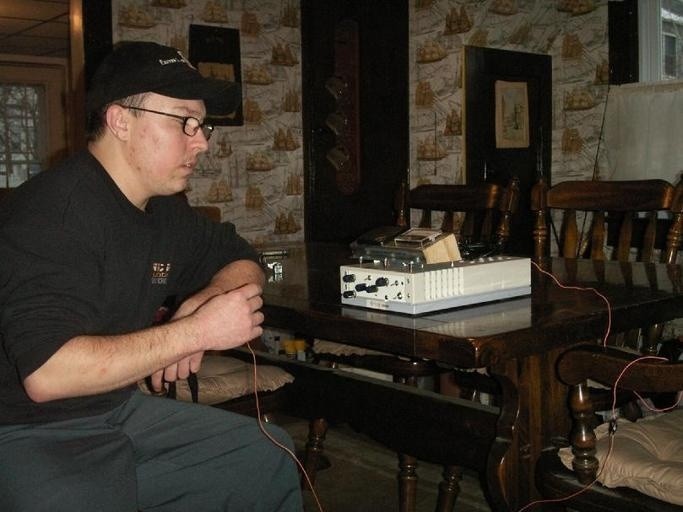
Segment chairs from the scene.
[391,167,522,256]
[526,178,681,356]
[300,347,523,509]
[531,334,683,512]
[137,206,296,418]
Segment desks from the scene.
[258,256,682,512]
[165,242,683,512]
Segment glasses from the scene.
[123,106,214,137]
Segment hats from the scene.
[84,38,242,115]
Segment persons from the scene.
[0,41,309,512]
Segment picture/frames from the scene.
[495,80,529,149]
